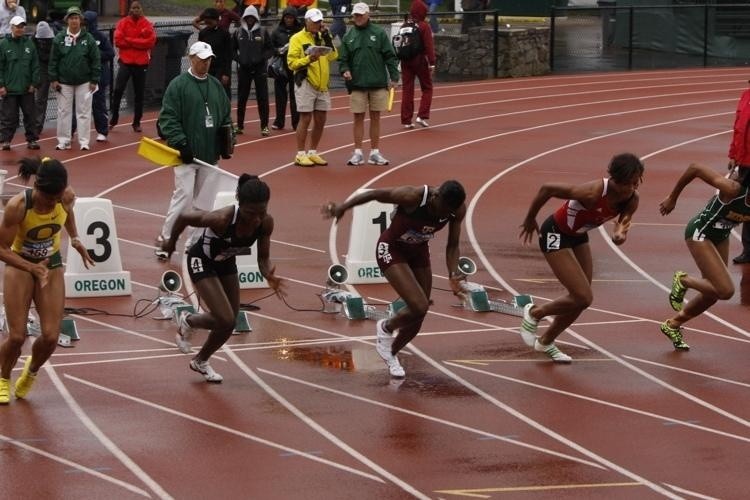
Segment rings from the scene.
[328,205,332,211]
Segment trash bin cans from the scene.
[127,30,193,110]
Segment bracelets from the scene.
[71,237,80,242]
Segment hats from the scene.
[9,15,27,26]
[63,6,85,24]
[200,8,219,20]
[188,41,217,60]
[304,8,325,23]
[350,2,370,15]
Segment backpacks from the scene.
[391,18,423,61]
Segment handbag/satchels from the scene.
[267,54,289,82]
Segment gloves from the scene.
[173,137,194,164]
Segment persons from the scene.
[154,40,236,260]
[151,173,288,382]
[657,162,749,351]
[398,1,464,129]
[517,151,645,362]
[728,87,750,265]
[1,0,155,151]
[317,179,468,379]
[0,155,97,406]
[192,0,399,165]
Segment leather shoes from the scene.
[732,253,750,264]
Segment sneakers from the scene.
[405,116,429,129]
[520,303,537,348]
[80,144,90,150]
[96,133,106,141]
[534,337,573,363]
[294,154,328,167]
[108,119,118,130]
[55,143,72,150]
[259,124,281,134]
[2,141,11,151]
[346,152,389,166]
[660,318,691,348]
[174,309,194,355]
[26,140,41,150]
[384,355,406,377]
[14,354,38,399]
[0,377,11,405]
[188,358,224,382]
[668,270,689,313]
[132,123,142,132]
[233,127,244,135]
[375,318,394,362]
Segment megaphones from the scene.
[327,264,349,286]
[457,257,476,280]
[160,270,182,293]
